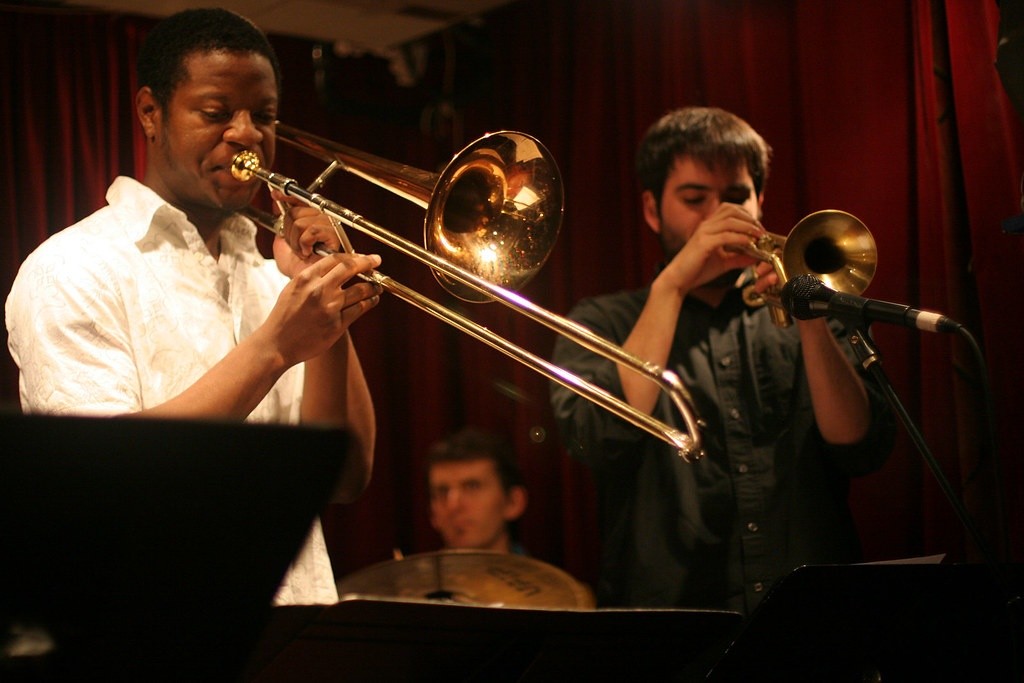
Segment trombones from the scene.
[233,119,709,463]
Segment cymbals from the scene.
[337,547,599,612]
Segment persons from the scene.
[5,7,385,606]
[426,427,531,550]
[547,107,901,629]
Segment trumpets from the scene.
[737,209,879,327]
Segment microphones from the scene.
[779,274,962,334]
